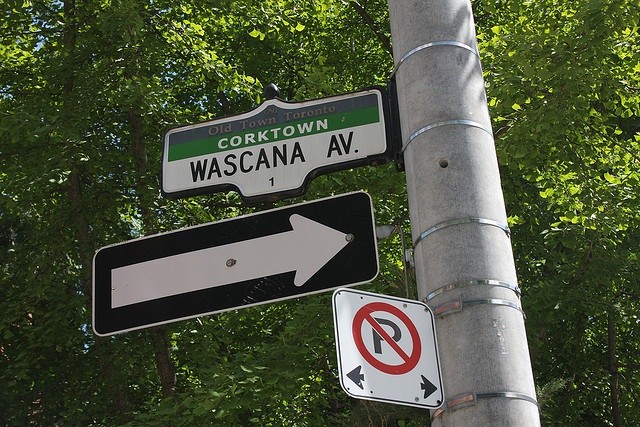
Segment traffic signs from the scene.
[330,287,444,409]
